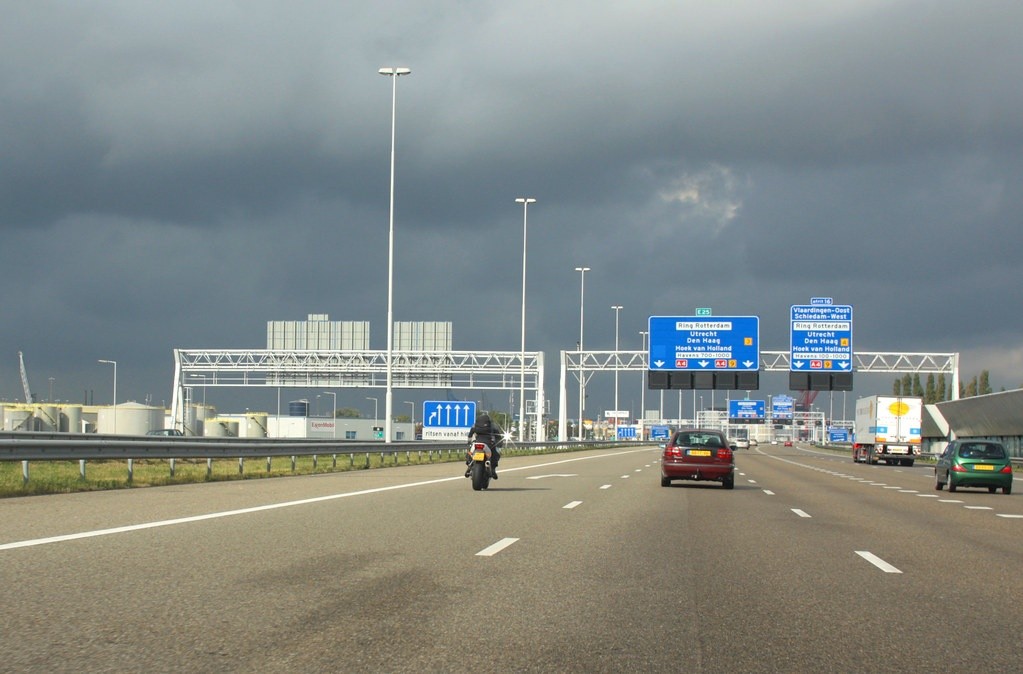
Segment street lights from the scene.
[815,408,820,441]
[639,332,649,445]
[792,399,797,441]
[515,198,536,442]
[576,267,591,442]
[611,306,623,445]
[324,392,336,439]
[190,374,206,436]
[404,401,414,440]
[367,397,378,427]
[99,359,117,434]
[767,394,772,442]
[745,390,751,440]
[379,66,411,443]
[810,403,813,441]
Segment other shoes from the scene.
[491,469,498,480]
[465,466,472,478]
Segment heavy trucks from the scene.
[853,394,922,466]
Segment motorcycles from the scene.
[466,438,496,490]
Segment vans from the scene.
[750,439,757,446]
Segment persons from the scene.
[463,413,502,479]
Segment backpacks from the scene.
[475,413,492,435]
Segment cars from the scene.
[784,442,792,446]
[935,439,1013,494]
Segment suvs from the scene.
[661,429,738,490]
[736,438,749,449]
